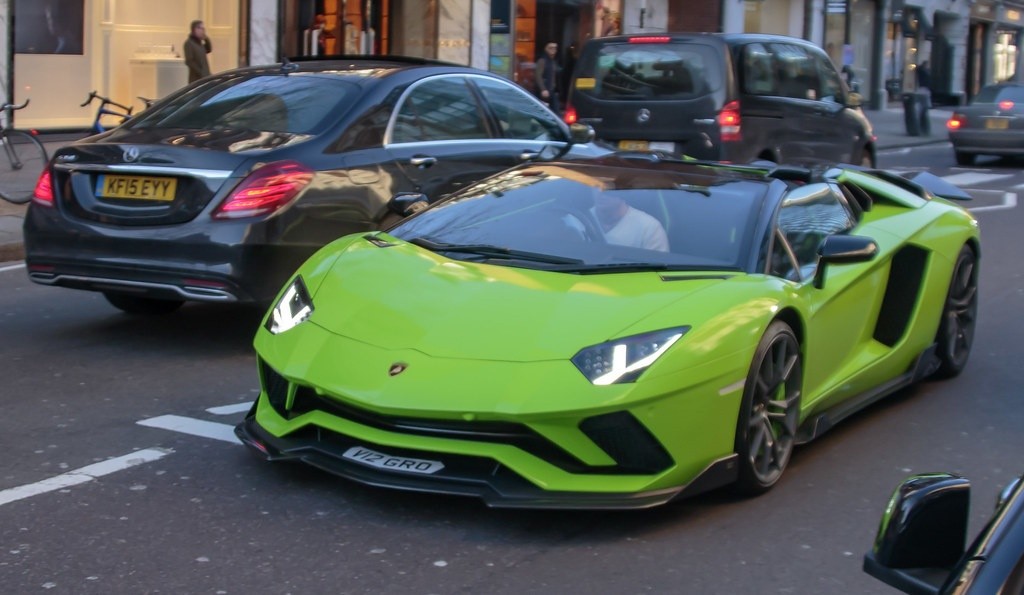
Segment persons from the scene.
[183,20,212,86]
[533,42,578,115]
[310,15,335,56]
[541,158,669,251]
[917,61,932,110]
[50,24,75,52]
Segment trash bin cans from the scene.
[902,93,930,135]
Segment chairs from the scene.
[604,58,693,98]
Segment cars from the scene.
[19,52,644,333]
[945,83,1024,163]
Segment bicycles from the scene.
[79,90,161,138]
[0,99,53,206]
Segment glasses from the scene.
[197,26,205,30]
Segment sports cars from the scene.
[231,142,984,520]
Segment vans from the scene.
[559,30,878,173]
